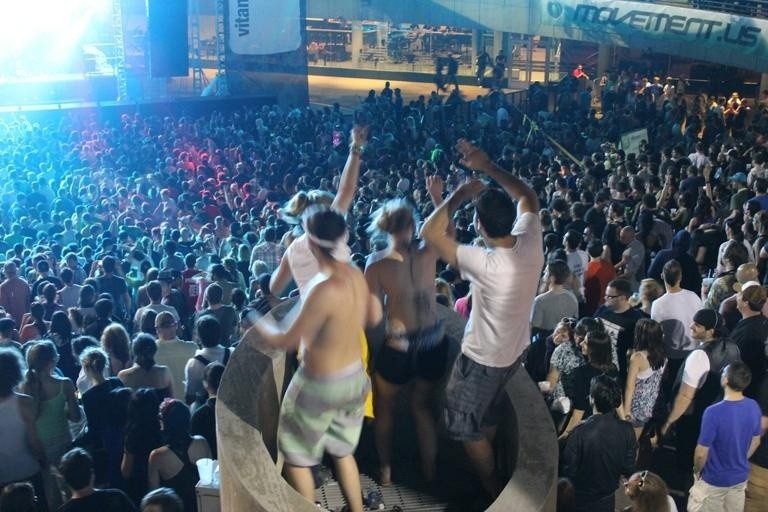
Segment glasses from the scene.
[561,317,576,324]
[639,471,647,489]
[607,296,619,300]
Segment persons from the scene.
[1,40,767,512]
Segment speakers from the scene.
[148,1,189,78]
